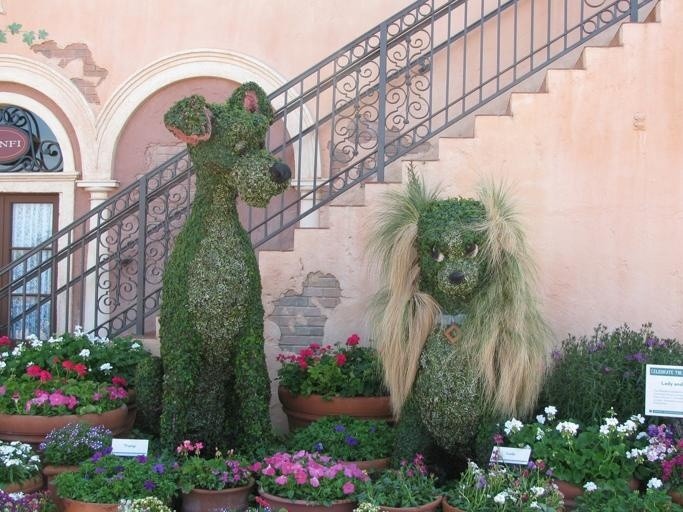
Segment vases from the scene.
[0,402,137,442]
[278,388,391,430]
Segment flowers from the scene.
[276,334,388,397]
[443,322,681,511]
[1,326,149,415]
[1,417,441,509]
